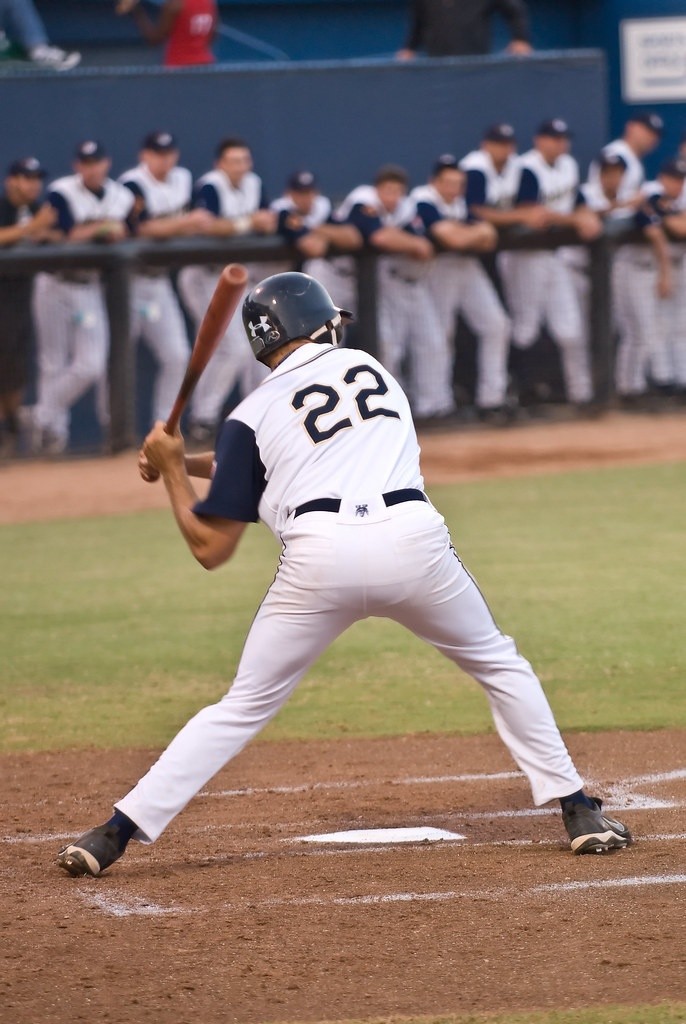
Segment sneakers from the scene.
[56,825,123,878]
[561,797,630,854]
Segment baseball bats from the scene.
[140,262,250,483]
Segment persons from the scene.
[116,0,219,67]
[0,112,686,455]
[397,0,533,59]
[0,0,82,71]
[55,270,631,874]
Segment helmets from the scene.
[241,272,353,367]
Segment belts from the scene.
[294,489,426,518]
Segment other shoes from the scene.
[32,46,81,70]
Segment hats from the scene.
[536,119,570,135]
[429,154,458,171]
[484,124,514,140]
[75,140,104,161]
[143,132,178,151]
[287,170,318,189]
[10,157,48,177]
[373,165,409,185]
[635,114,666,136]
